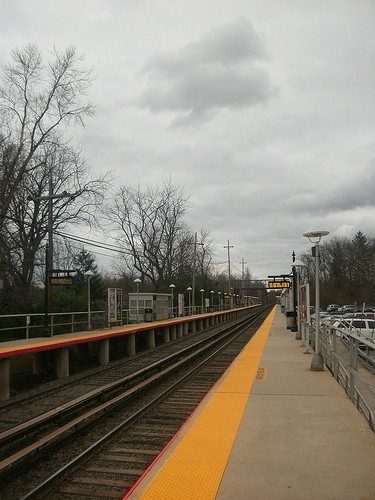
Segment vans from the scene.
[310,312,375,346]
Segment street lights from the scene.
[187,286,192,316]
[218,292,222,311]
[84,269,95,330]
[210,291,214,312]
[133,277,142,323]
[303,230,330,371]
[200,288,204,313]
[169,283,176,319]
[290,259,305,339]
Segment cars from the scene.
[355,305,375,312]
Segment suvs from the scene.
[327,304,340,312]
[338,305,355,313]
